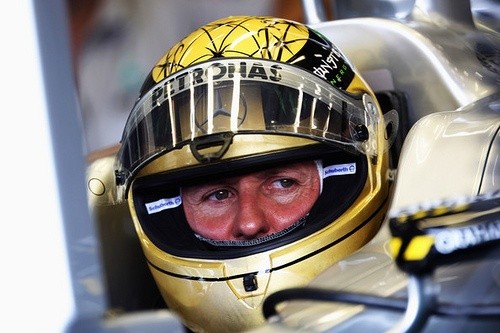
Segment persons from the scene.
[115,13,394,333]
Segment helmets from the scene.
[113,16,390,333]
